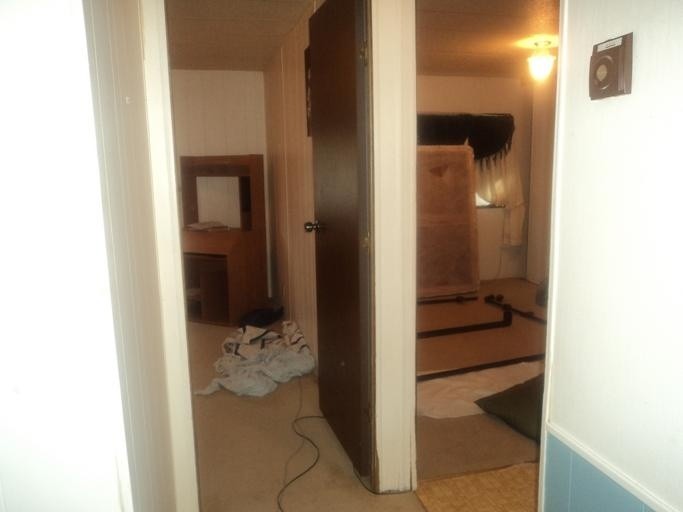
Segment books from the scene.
[187,221,224,232]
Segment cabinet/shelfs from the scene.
[176,153,266,328]
[415,140,482,302]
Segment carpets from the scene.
[415,356,544,483]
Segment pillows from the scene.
[474,372,544,443]
